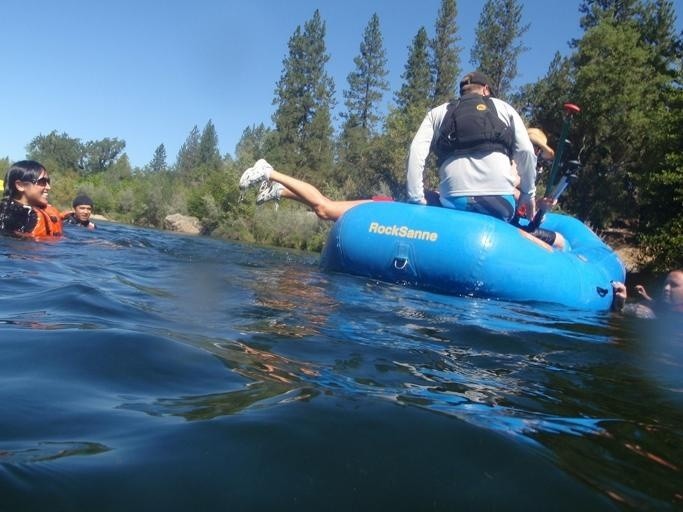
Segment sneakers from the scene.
[256,179,285,205]
[239,159,273,190]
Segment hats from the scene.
[460,71,497,97]
[73,195,94,208]
[528,128,555,160]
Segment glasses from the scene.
[22,176,51,187]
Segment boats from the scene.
[318,211,625,313]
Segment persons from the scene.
[0,160,127,248]
[239,159,394,220]
[62,193,97,230]
[515,125,572,253]
[406,70,546,228]
[610,267,683,322]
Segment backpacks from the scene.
[432,93,514,165]
